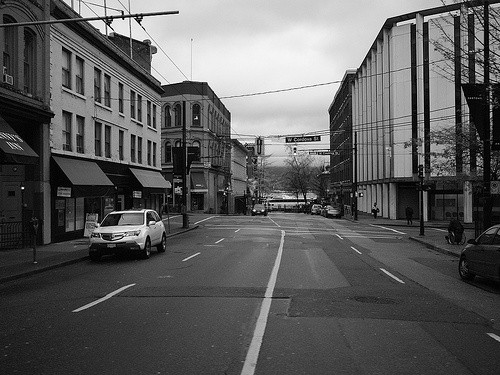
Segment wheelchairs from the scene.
[443,219,466,245]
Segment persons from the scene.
[372,202,380,219]
[445,218,458,240]
[178,197,182,213]
[405,205,414,224]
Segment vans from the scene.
[459,225,500,285]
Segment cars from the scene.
[311,204,322,215]
[251,203,268,216]
[321,205,342,219]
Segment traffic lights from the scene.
[418,164,424,180]
[293,147,297,156]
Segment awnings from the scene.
[0,117,172,188]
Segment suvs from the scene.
[88,209,168,260]
